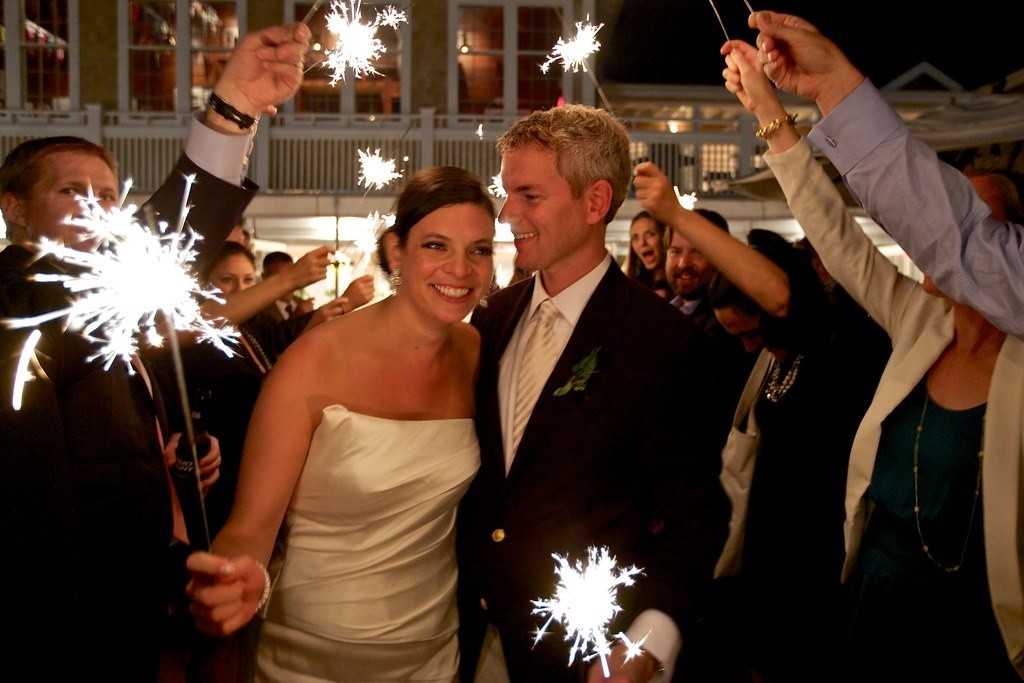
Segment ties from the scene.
[286,305,298,319]
[512,301,560,464]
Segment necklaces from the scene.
[914,382,985,574]
[764,356,803,401]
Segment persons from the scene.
[0,23,375,683]
[471,101,726,683]
[190,161,497,683]
[613,193,888,683]
[749,8,1024,335]
[720,42,1023,683]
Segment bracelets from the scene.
[256,558,271,612]
[207,92,258,130]
[753,116,796,140]
